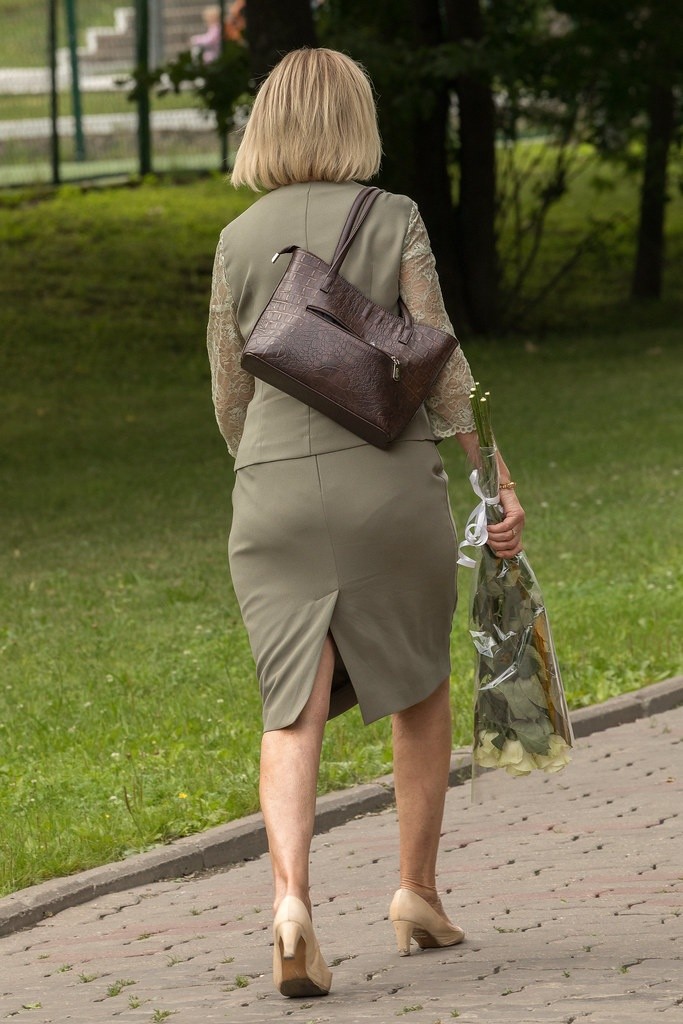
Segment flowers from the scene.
[468,381,573,778]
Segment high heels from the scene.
[272,895,333,998]
[389,889,465,957]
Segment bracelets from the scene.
[499,482,517,489]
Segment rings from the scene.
[512,529,516,537]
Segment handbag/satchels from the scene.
[242,187,459,451]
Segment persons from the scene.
[205,48,524,996]
